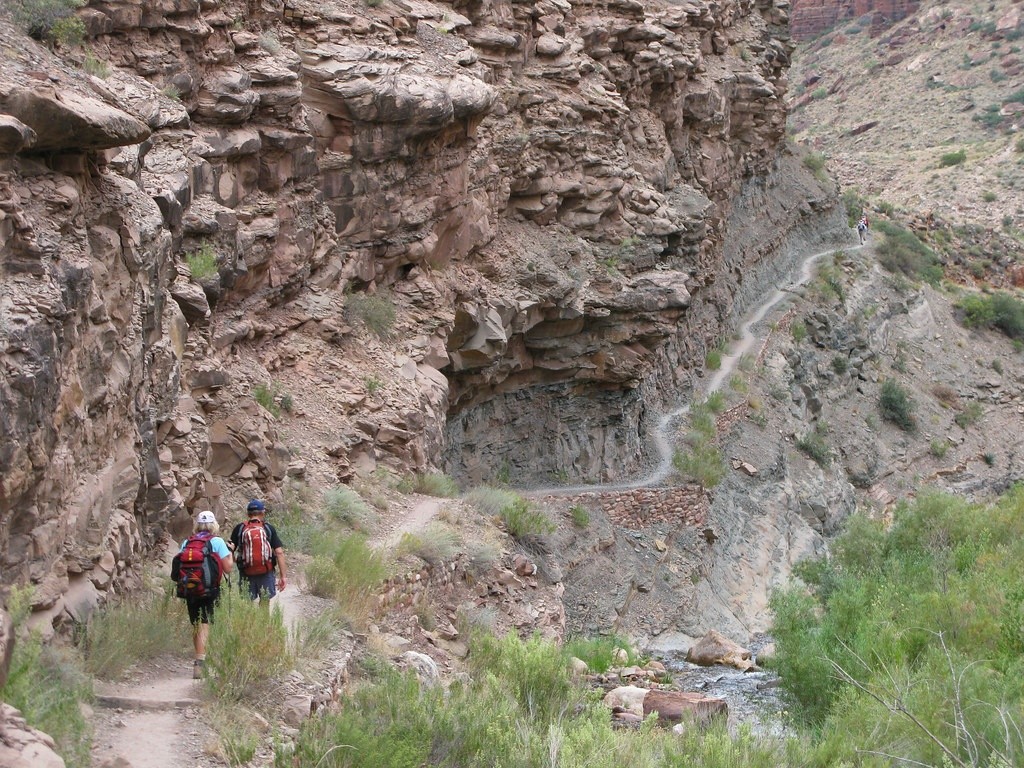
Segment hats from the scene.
[196,510,215,523]
[247,499,263,510]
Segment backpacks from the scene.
[236,520,277,594]
[171,535,228,605]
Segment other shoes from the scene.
[192,661,210,679]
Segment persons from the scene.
[171,511,234,679]
[858,218,868,245]
[227,500,286,610]
